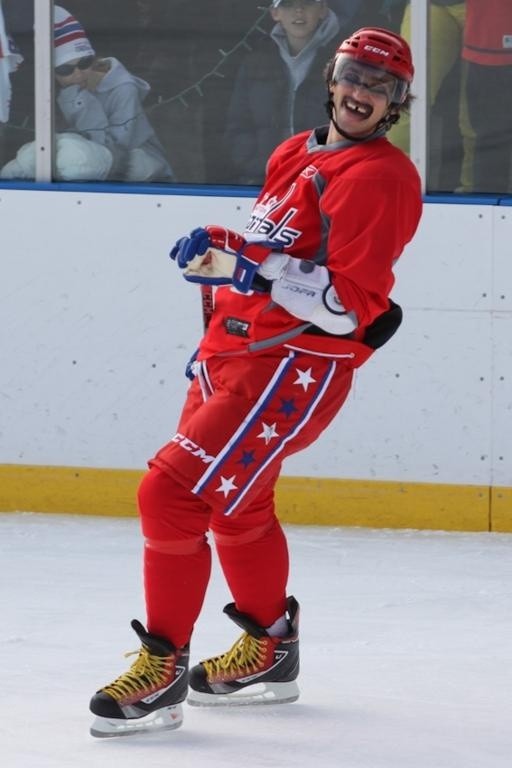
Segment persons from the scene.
[0,0,180,182]
[432,0,512,193]
[88,25,427,720]
[225,0,354,184]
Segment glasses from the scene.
[53,55,95,76]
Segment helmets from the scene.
[336,26,415,103]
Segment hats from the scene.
[32,4,95,70]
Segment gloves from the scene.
[168,224,285,294]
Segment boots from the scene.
[188,594,301,696]
[90,618,189,720]
